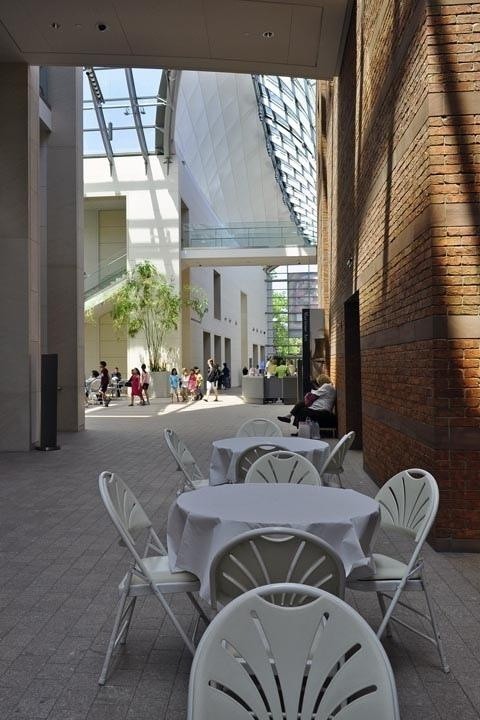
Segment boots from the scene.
[195,393,203,401]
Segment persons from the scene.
[85,354,297,408]
[276,374,338,436]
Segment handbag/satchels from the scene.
[303,392,318,407]
[143,383,149,389]
[125,381,131,386]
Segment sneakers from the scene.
[277,416,292,423]
[104,399,111,407]
[128,401,151,406]
[290,432,298,436]
[204,399,218,402]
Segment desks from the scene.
[198,436,333,485]
[162,481,383,606]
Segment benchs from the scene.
[315,426,337,438]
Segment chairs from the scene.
[159,426,214,494]
[314,428,360,488]
[186,582,401,718]
[199,526,350,711]
[245,450,325,486]
[232,443,291,485]
[96,466,214,687]
[347,468,454,675]
[235,417,287,440]
[88,379,103,406]
